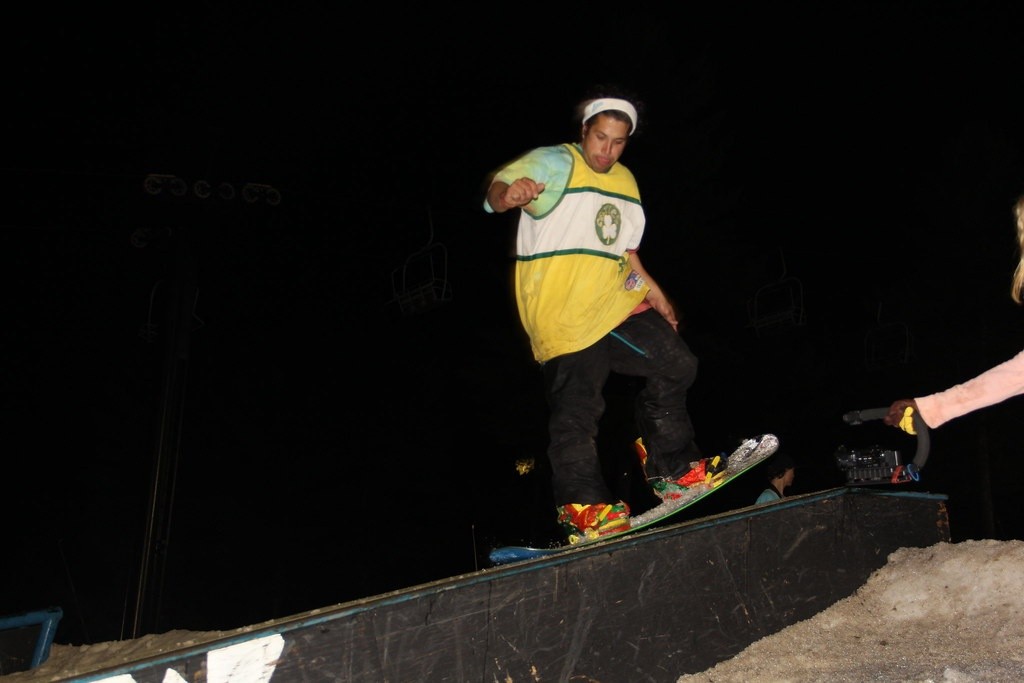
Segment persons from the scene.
[755,454,796,505]
[885,346,1023,433]
[483,98,729,544]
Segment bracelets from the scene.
[500,192,515,209]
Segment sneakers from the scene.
[652,450,728,505]
[555,498,632,546]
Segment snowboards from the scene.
[488,434,779,566]
